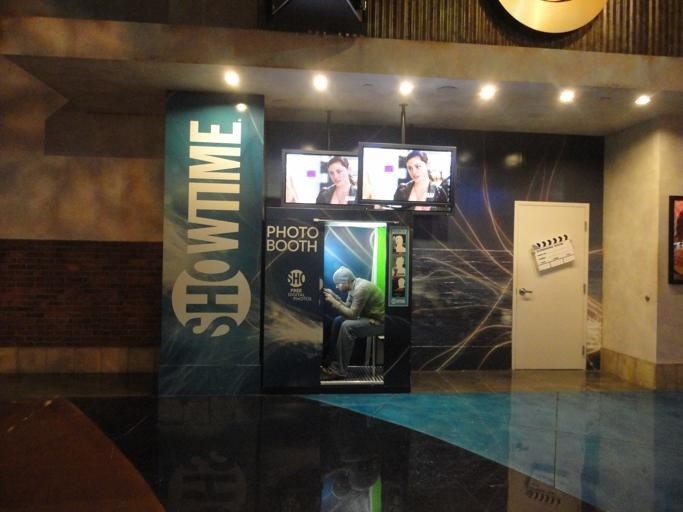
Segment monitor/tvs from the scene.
[358,142,456,205]
[281,149,374,210]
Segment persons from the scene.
[394,150,447,201]
[316,155,357,204]
[321,266,384,379]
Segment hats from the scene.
[333,267,355,284]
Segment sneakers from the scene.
[321,365,347,378]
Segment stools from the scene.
[364,334,385,377]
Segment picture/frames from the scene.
[667,194,682,285]
